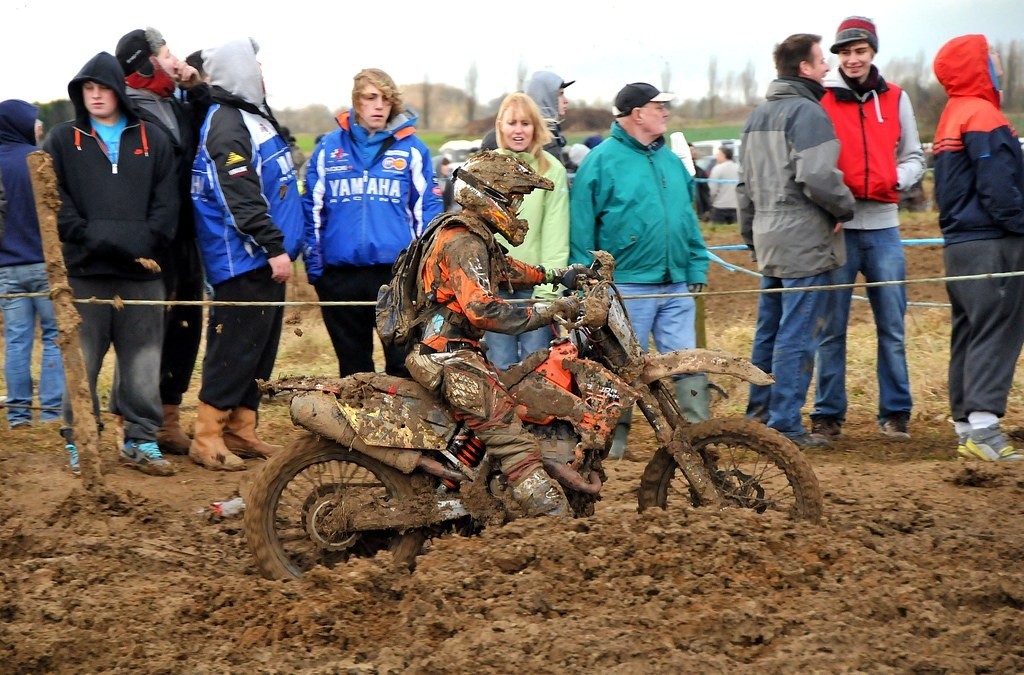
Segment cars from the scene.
[693,139,741,159]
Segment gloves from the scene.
[552,264,604,290]
[543,297,580,322]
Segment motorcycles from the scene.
[244,249,822,583]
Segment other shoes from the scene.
[790,434,828,447]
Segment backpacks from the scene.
[376,210,457,350]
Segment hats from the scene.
[612,82,677,118]
[115,28,187,118]
[830,16,879,54]
[561,80,575,88]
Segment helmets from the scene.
[453,151,554,247]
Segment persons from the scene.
[931,33,1024,466]
[0,27,309,474]
[434,71,604,176]
[809,15,926,445]
[735,33,858,449]
[300,67,446,378]
[680,141,742,223]
[482,91,570,368]
[403,153,603,516]
[571,83,719,460]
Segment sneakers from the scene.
[812,418,842,440]
[65,443,81,475]
[958,423,1024,462]
[878,417,912,439]
[120,441,175,475]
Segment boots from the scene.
[673,377,729,424]
[609,407,633,461]
[189,401,248,471]
[157,405,192,455]
[222,407,284,459]
